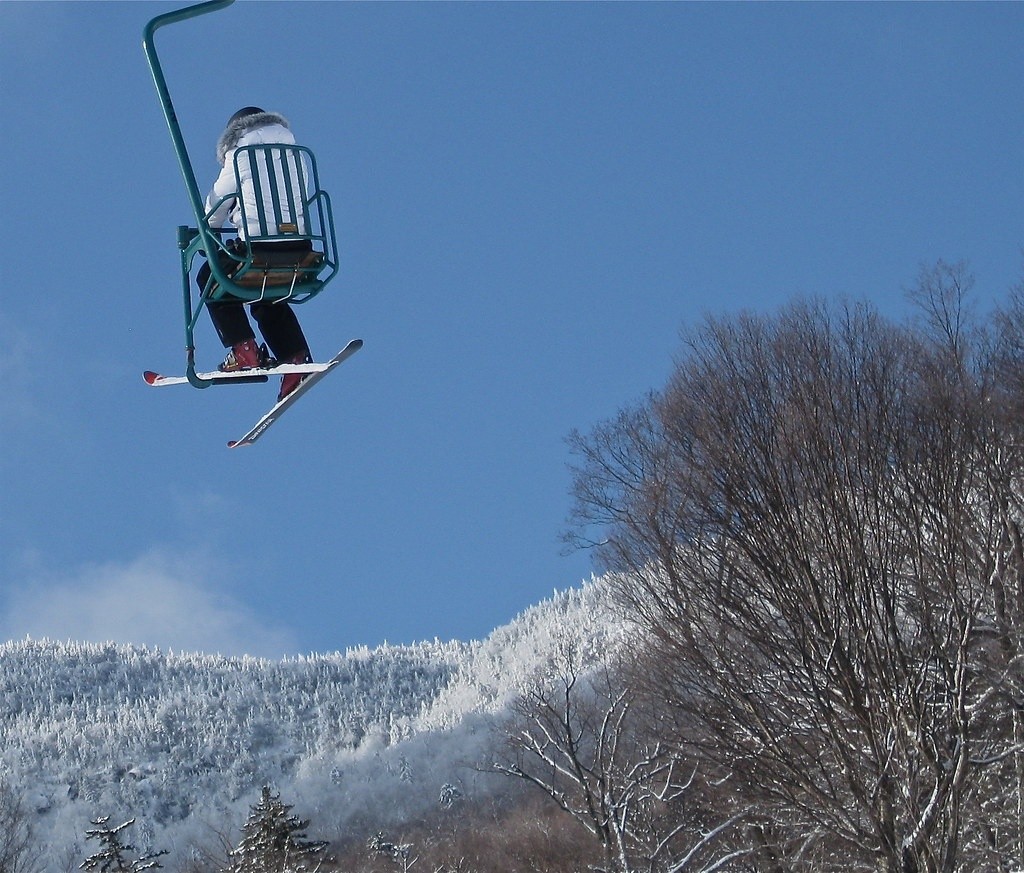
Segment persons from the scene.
[195,107,314,403]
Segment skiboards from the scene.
[140,336,364,450]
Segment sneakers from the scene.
[277,353,310,402]
[217,338,261,372]
